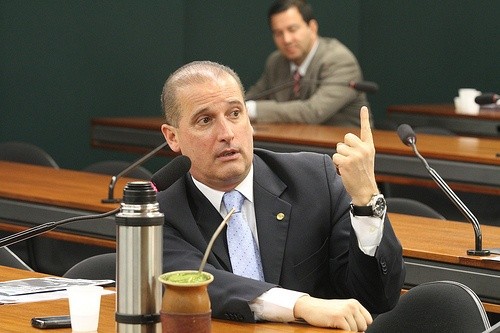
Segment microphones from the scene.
[99,79,380,206]
[474,92,500,105]
[397,123,489,256]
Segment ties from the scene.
[223,191,265,282]
[292,69,301,97]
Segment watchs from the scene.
[349,193,387,218]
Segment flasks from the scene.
[115,181,165,333]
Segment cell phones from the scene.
[30,314,71,329]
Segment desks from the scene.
[0,104,500,333]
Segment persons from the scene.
[245,0,374,129]
[151,61,406,331]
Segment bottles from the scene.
[158,269,214,333]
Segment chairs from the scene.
[61,252,117,283]
[0,141,59,170]
[82,160,154,182]
[382,197,446,221]
[365,280,492,333]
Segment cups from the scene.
[453,88,481,115]
[67,284,103,332]
